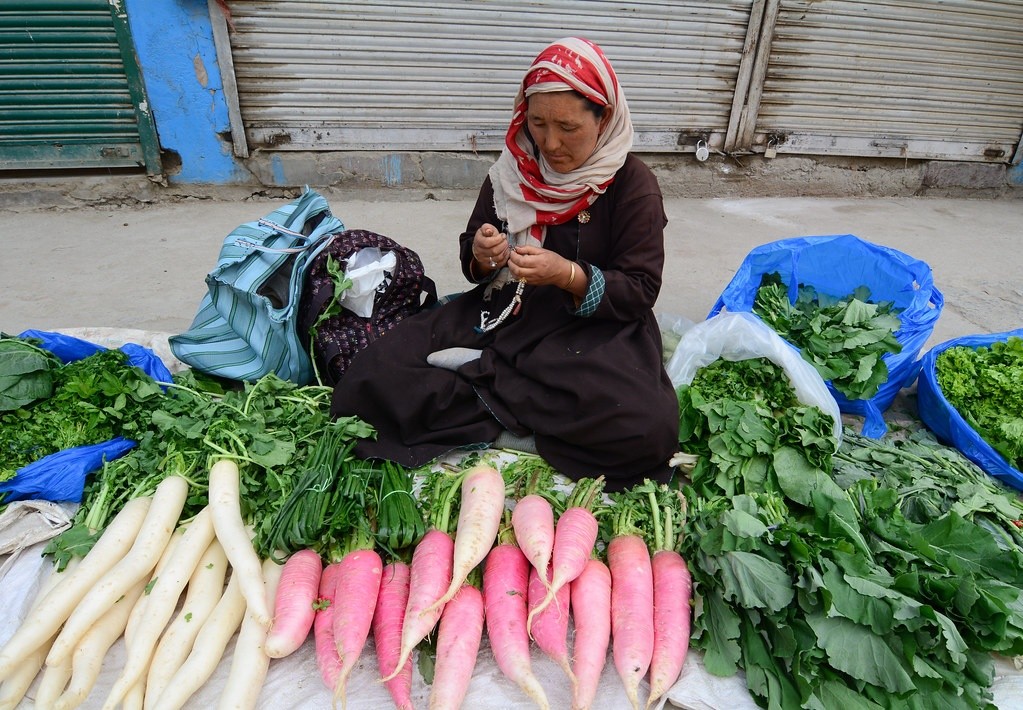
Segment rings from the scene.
[518,276,527,285]
[488,256,497,267]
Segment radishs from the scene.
[1,373,693,710]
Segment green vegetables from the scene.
[309,249,352,384]
[669,271,1023,710]
[1,332,165,483]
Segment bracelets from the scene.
[560,261,575,291]
[471,242,479,263]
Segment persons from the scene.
[326,37,680,498]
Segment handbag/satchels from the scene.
[705,233,944,440]
[664,312,844,455]
[296,227,439,387]
[919,327,1022,490]
[167,182,343,391]
